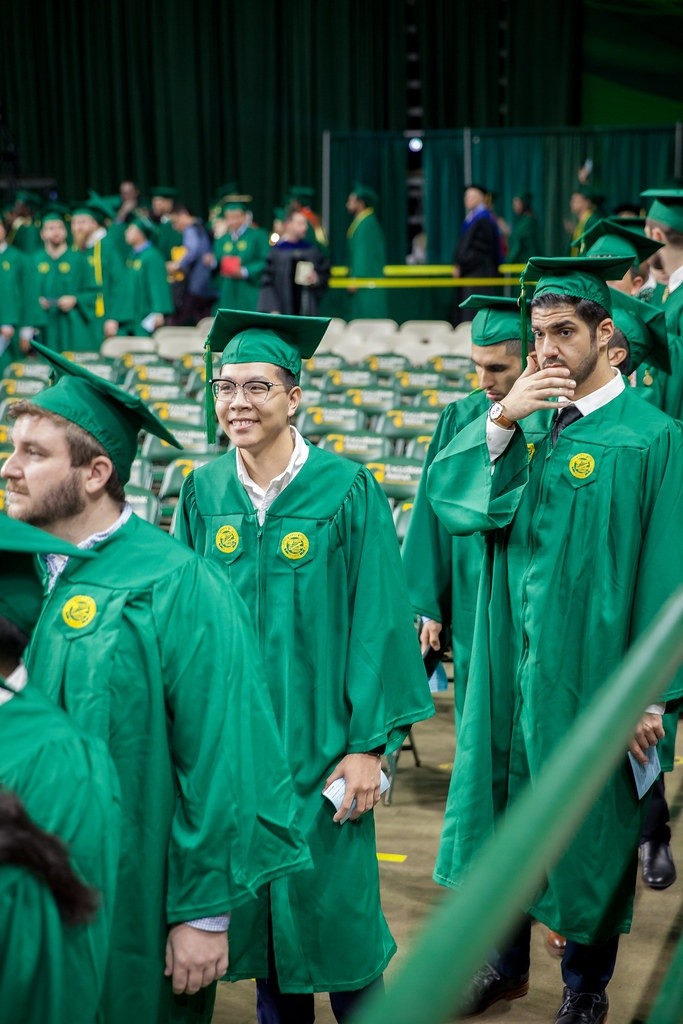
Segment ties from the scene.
[552,407,585,450]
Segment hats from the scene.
[288,186,314,205]
[28,339,184,487]
[217,184,251,211]
[204,309,332,444]
[15,187,176,234]
[0,510,100,637]
[354,182,375,199]
[458,257,672,376]
[571,184,683,271]
[512,186,533,206]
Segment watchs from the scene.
[489,402,515,428]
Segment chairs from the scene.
[0,318,474,552]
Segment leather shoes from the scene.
[555,986,609,1024]
[546,932,566,955]
[640,842,676,889]
[455,962,529,1020]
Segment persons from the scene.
[595,283,680,890]
[0,339,311,1024]
[0,785,101,1024]
[570,219,683,423]
[453,167,683,334]
[426,255,683,1024]
[344,182,390,320]
[0,180,330,368]
[172,307,436,1024]
[399,294,575,954]
[0,510,122,1024]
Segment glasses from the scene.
[209,379,293,403]
